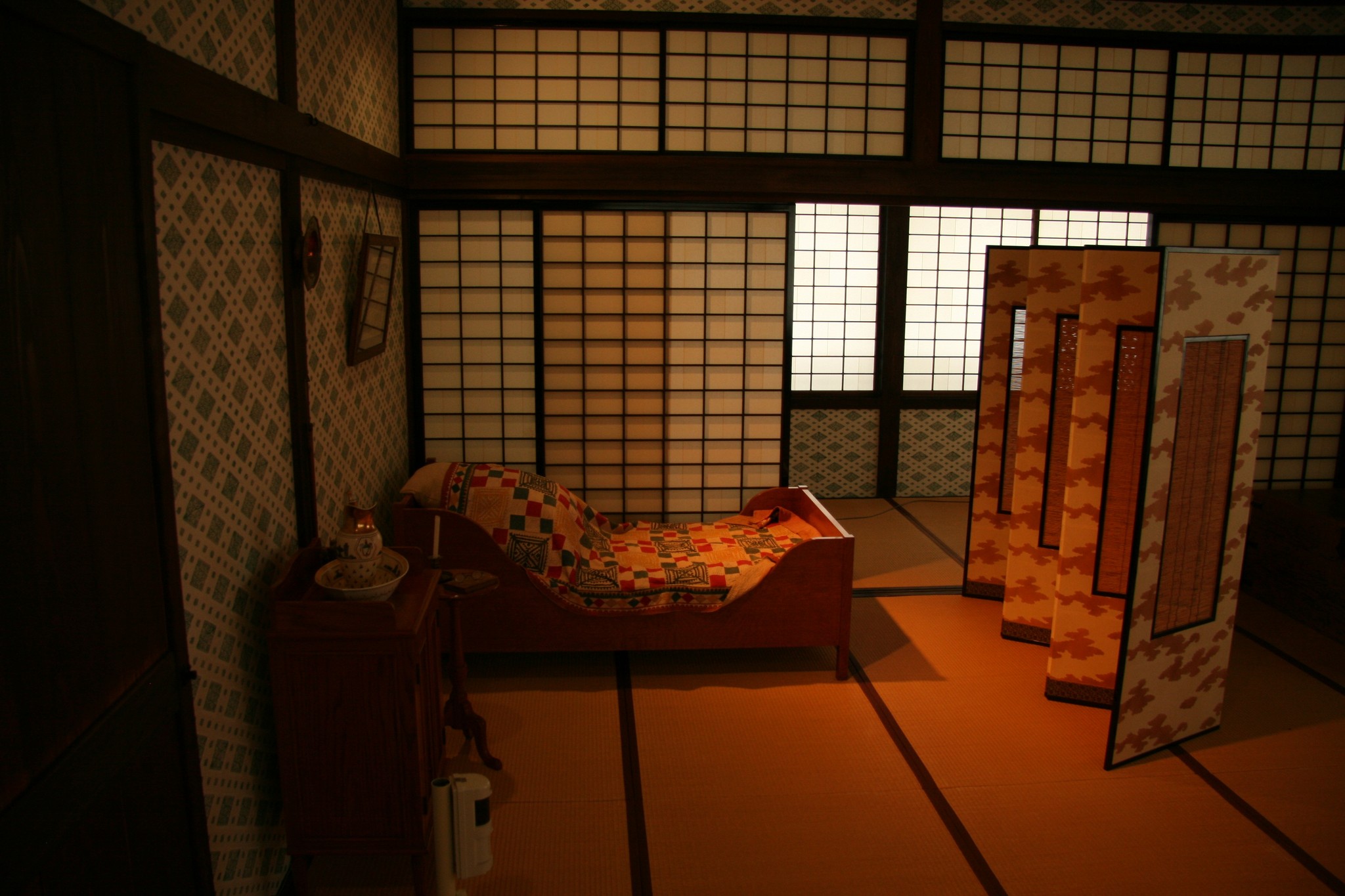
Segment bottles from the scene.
[334,485,382,587]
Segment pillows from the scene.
[399,463,452,507]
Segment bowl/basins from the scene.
[315,547,409,601]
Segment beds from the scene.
[393,485,855,683]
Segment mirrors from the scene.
[346,233,399,366]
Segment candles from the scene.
[432,515,440,557]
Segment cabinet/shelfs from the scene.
[263,537,444,896]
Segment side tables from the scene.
[437,568,503,770]
